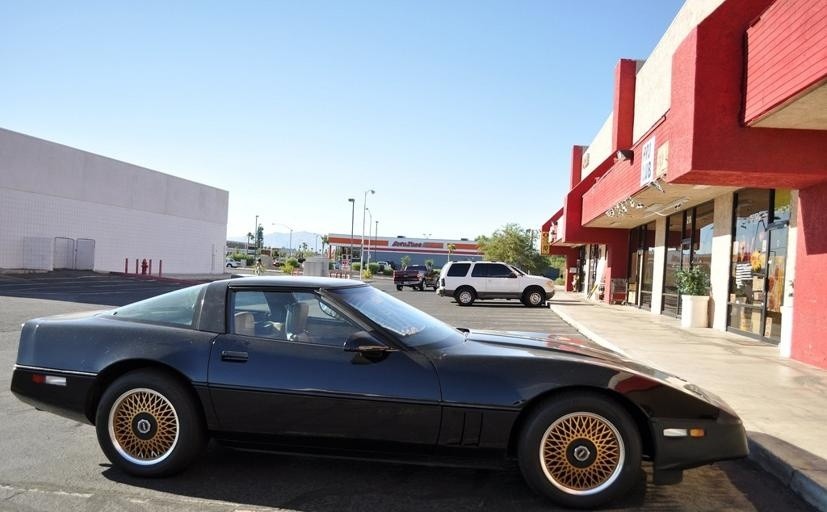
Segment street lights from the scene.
[315,234,323,256]
[255,215,259,256]
[272,222,293,256]
[359,189,379,280]
[349,198,355,254]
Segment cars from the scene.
[226,258,241,268]
[8,276,750,508]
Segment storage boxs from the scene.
[730,274,781,341]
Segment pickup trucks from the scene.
[393,266,440,291]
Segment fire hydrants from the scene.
[141,259,148,274]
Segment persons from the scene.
[253,257,264,276]
[335,258,352,274]
[271,258,285,270]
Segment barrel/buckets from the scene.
[680,295,710,329]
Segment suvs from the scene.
[377,261,389,272]
[437,260,556,307]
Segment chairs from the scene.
[234,311,257,336]
[285,302,313,343]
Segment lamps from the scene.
[616,150,635,161]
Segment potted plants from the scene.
[676,269,709,329]
